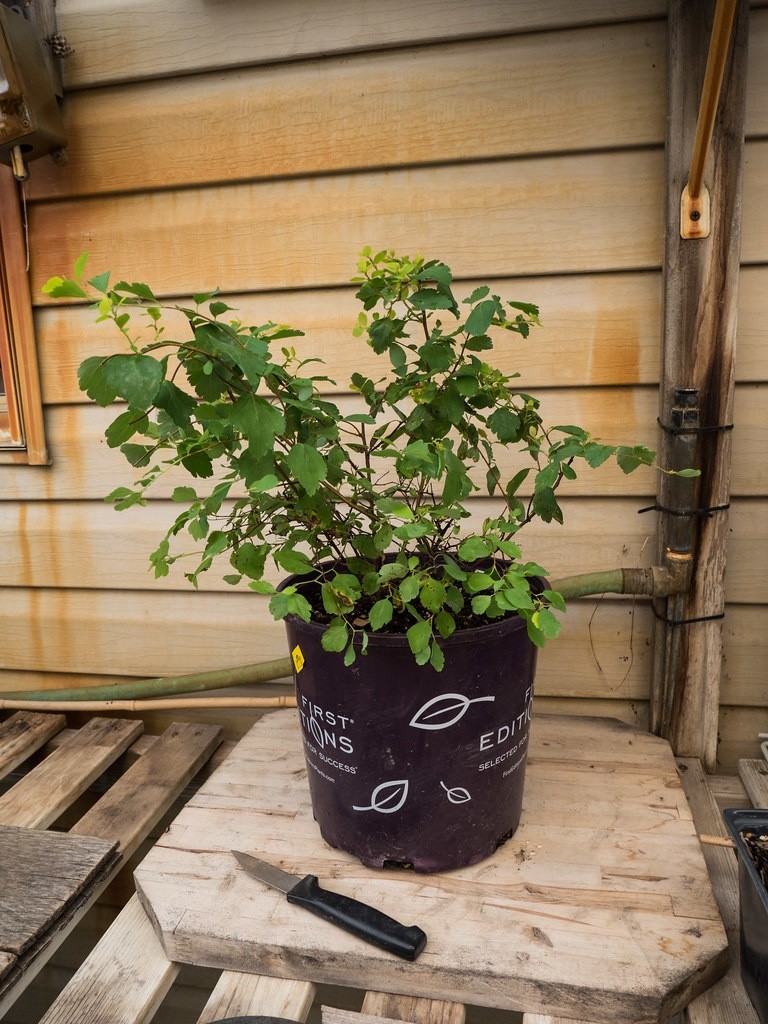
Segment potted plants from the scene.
[41,244,703,871]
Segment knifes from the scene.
[232,850,427,962]
